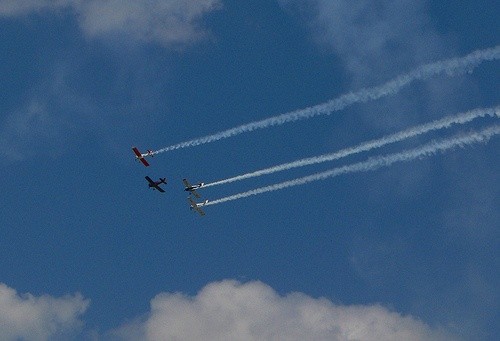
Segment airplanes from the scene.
[187,195,209,217]
[145,175,167,193]
[181,177,205,199]
[128,144,154,168]
